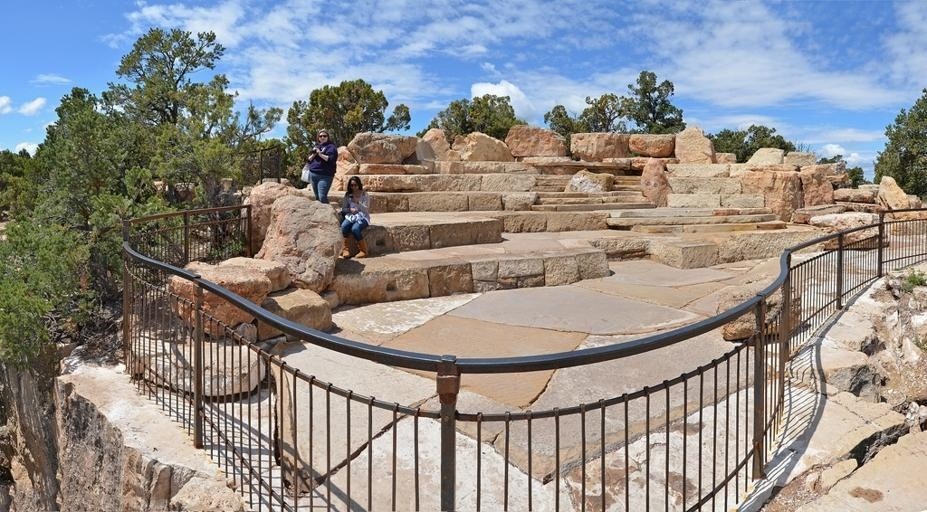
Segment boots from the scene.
[355,239,368,258]
[343,240,351,257]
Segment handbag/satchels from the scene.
[345,211,365,224]
[300,165,310,184]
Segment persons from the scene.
[307,128,337,205]
[338,176,371,258]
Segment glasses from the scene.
[319,135,327,137]
